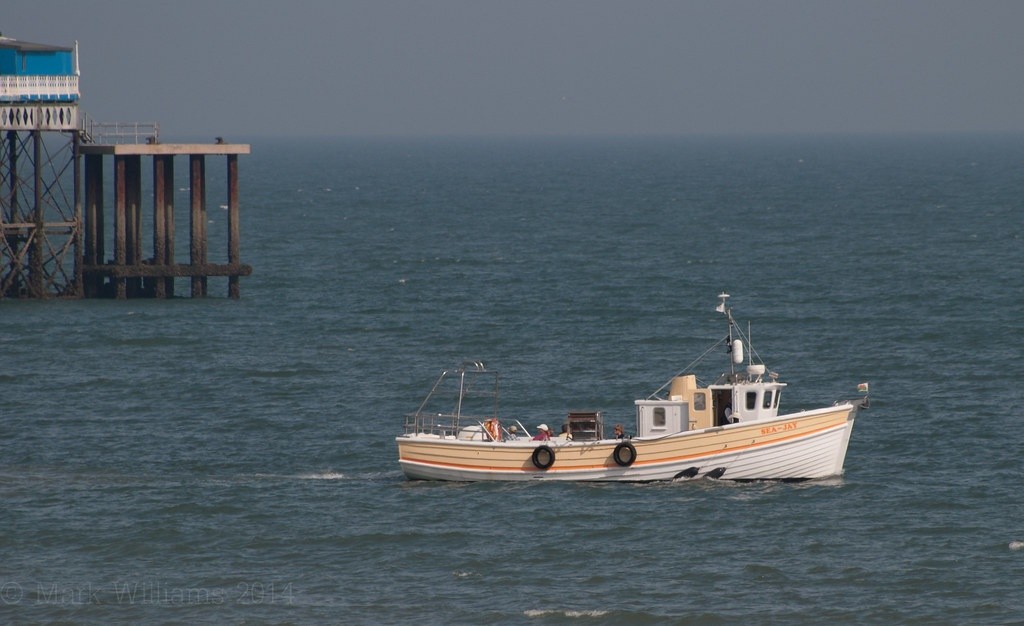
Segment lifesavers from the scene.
[532,445,555,469]
[614,442,637,467]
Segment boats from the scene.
[401,291,873,484]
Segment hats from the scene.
[537,424,548,431]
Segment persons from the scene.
[558,423,574,441]
[720,394,731,426]
[504,426,520,442]
[614,423,624,439]
[528,423,553,442]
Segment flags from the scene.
[856,382,868,392]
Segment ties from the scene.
[508,426,517,433]
